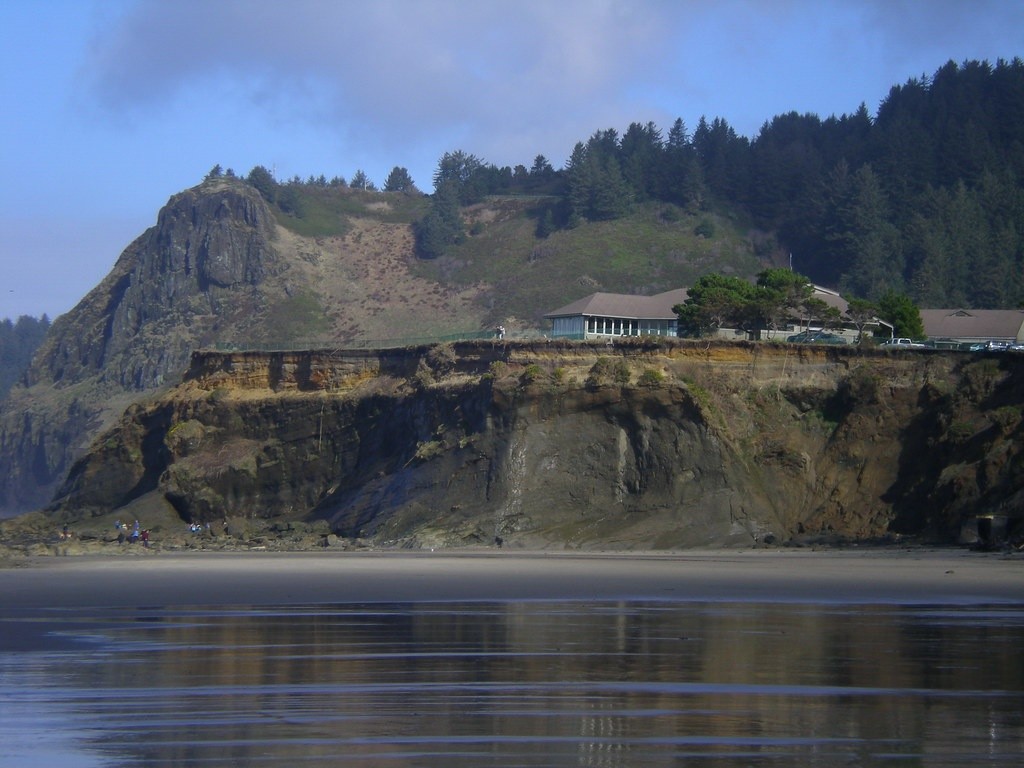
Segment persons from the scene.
[63,517,228,548]
[493,326,506,338]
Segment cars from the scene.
[970,340,1024,352]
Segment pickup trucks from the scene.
[786,329,847,345]
[878,337,925,348]
